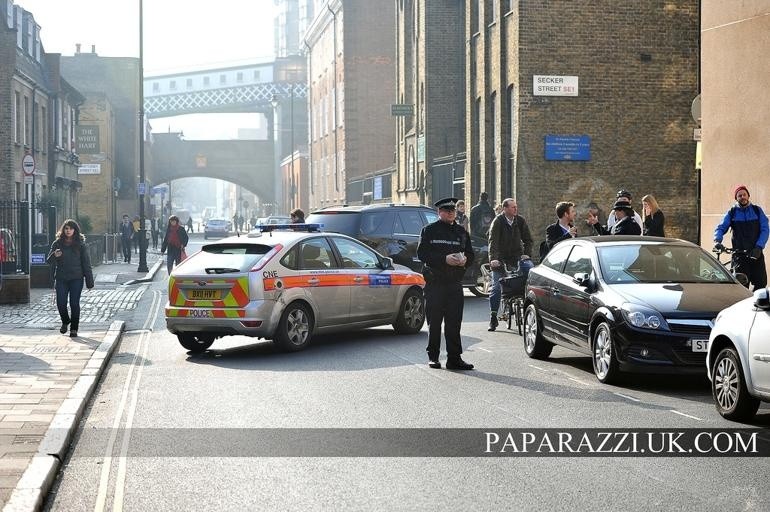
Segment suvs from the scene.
[306,200,494,300]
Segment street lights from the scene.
[268,84,296,212]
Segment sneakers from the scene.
[70,330,77,337]
[60,320,71,333]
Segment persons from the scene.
[415,196,476,370]
[487,198,534,330]
[46,219,94,337]
[118,212,194,277]
[233,212,257,233]
[713,186,769,289]
[290,210,303,226]
[545,200,580,245]
[585,189,665,237]
[454,193,504,237]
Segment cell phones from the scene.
[56,249,61,253]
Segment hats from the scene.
[612,200,632,210]
[735,185,750,200]
[434,197,458,209]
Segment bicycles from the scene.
[701,243,757,284]
[488,257,532,337]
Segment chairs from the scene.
[645,260,670,280]
[303,244,327,268]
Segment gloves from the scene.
[752,247,761,258]
[715,242,723,249]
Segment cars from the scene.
[166,222,427,352]
[704,286,770,421]
[0,226,19,277]
[176,207,233,240]
[522,236,754,386]
[252,215,296,231]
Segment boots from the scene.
[446,353,474,369]
[428,353,441,367]
[487,311,498,331]
